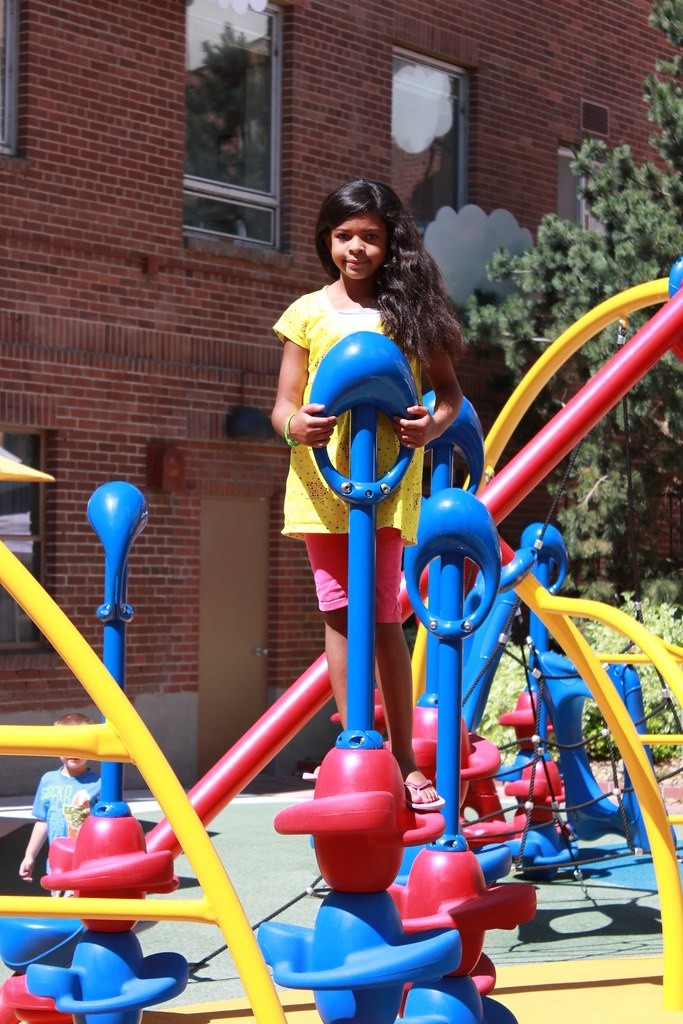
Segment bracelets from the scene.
[284,413,301,447]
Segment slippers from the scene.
[302,766,321,782]
[401,779,446,811]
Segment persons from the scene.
[18,712,101,899]
[267,179,468,809]
